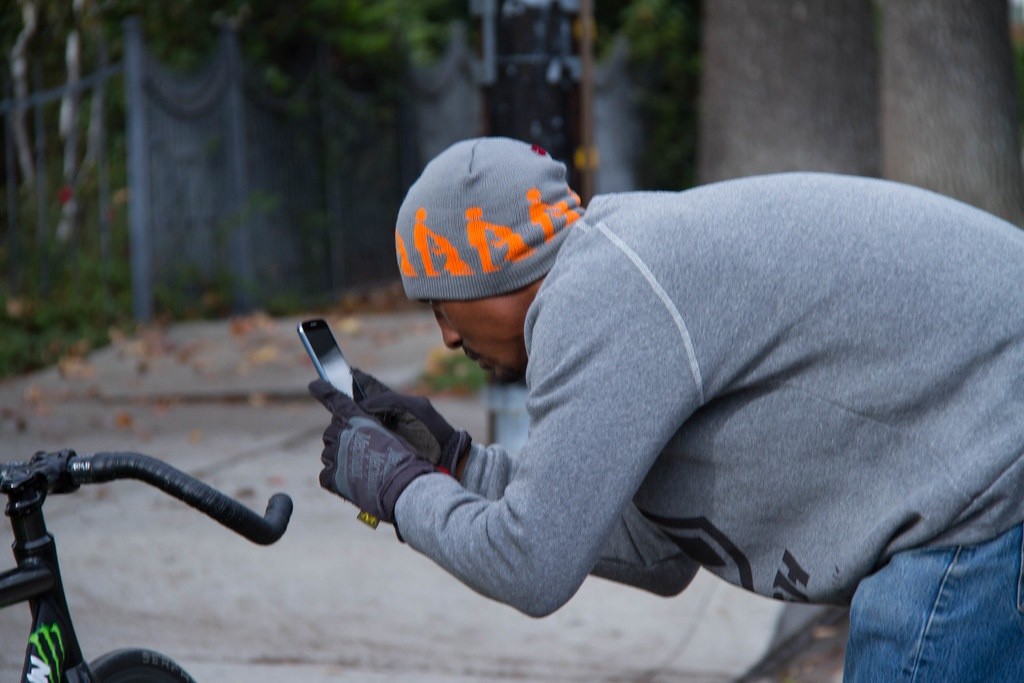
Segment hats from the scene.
[394,136,587,302]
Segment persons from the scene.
[307,136,1024,683]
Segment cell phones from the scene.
[297,318,367,408]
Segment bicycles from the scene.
[0,448,294,683]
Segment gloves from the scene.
[349,367,472,478]
[308,378,438,543]
